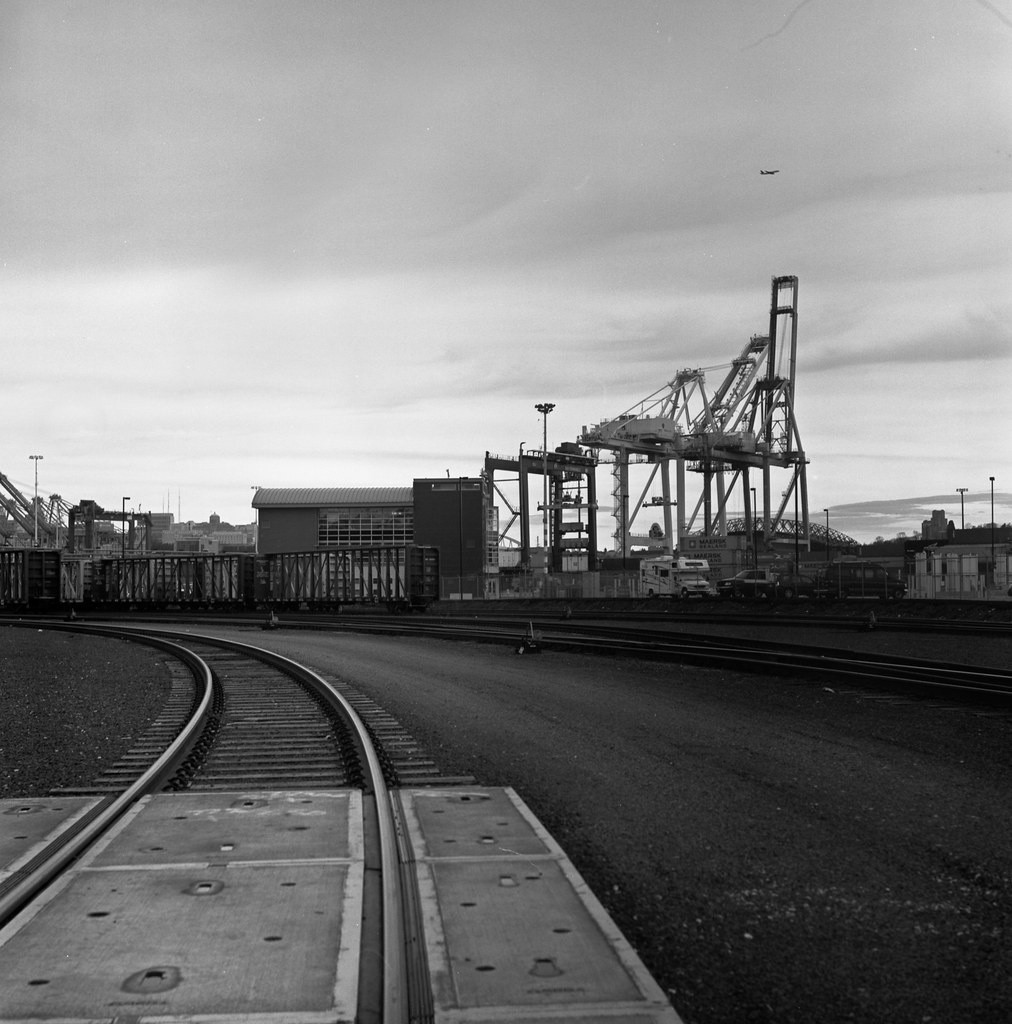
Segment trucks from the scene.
[640,555,711,597]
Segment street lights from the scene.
[824,508,829,561]
[534,402,556,572]
[785,456,810,575]
[956,488,968,529]
[250,485,262,553]
[28,454,44,547]
[750,487,757,570]
[459,476,469,600]
[990,476,995,568]
[122,497,131,559]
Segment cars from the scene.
[717,569,815,599]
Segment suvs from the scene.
[824,561,908,603]
